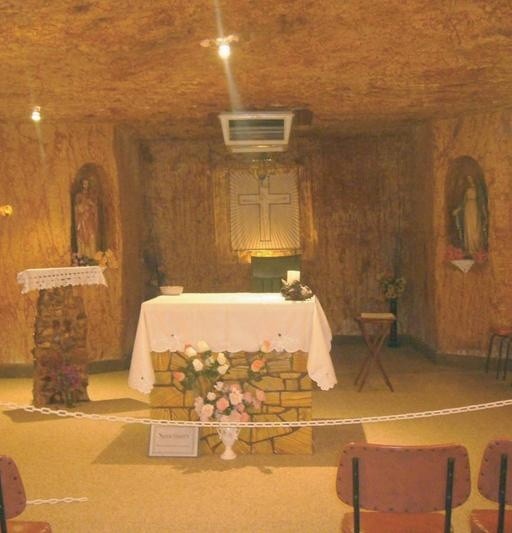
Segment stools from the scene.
[352,313,397,393]
[483,326,511,381]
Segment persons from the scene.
[75,178,101,262]
[451,168,489,255]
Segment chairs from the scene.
[0,456,52,532]
[334,434,511,533]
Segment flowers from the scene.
[172,335,276,423]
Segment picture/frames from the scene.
[149,424,200,457]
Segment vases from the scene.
[217,426,241,461]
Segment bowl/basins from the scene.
[159,285,185,296]
